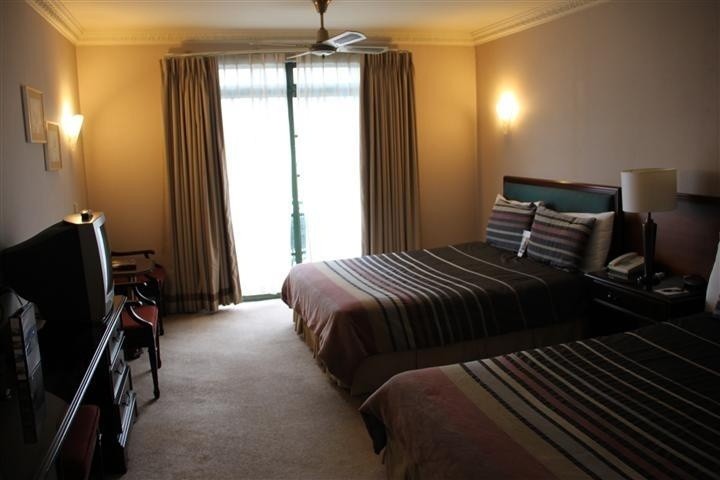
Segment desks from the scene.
[0,294,139,480]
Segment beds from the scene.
[282,174,622,399]
[361,241,719,479]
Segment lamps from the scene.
[621,167,677,285]
[496,94,517,138]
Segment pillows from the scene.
[495,193,545,209]
[556,211,615,273]
[526,205,596,274]
[485,199,537,253]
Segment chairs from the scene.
[114,282,161,401]
[110,249,169,336]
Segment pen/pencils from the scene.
[661,289,684,292]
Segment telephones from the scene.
[607,251,644,274]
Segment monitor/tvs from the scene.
[0,211,114,326]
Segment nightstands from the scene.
[584,262,709,341]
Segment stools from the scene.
[55,405,107,480]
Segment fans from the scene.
[249,0,389,61]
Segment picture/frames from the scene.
[44,121,63,172]
[23,84,48,144]
